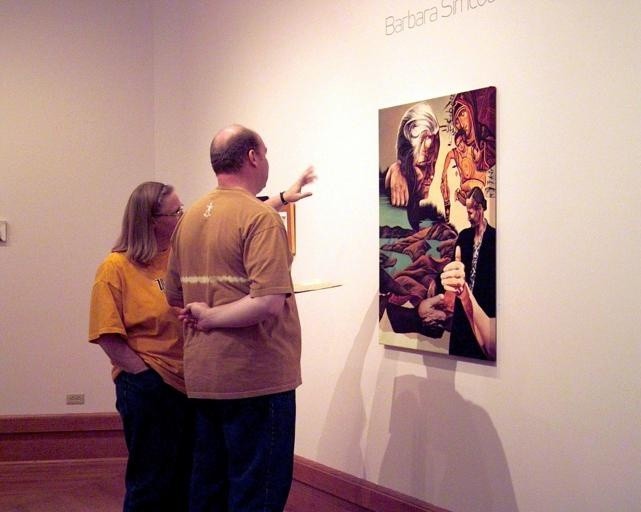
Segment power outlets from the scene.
[65,392,85,404]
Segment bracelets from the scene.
[279,190,290,206]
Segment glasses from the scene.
[157,211,181,220]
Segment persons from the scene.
[379,93,495,359]
[88,165,319,512]
[162,121,305,511]
